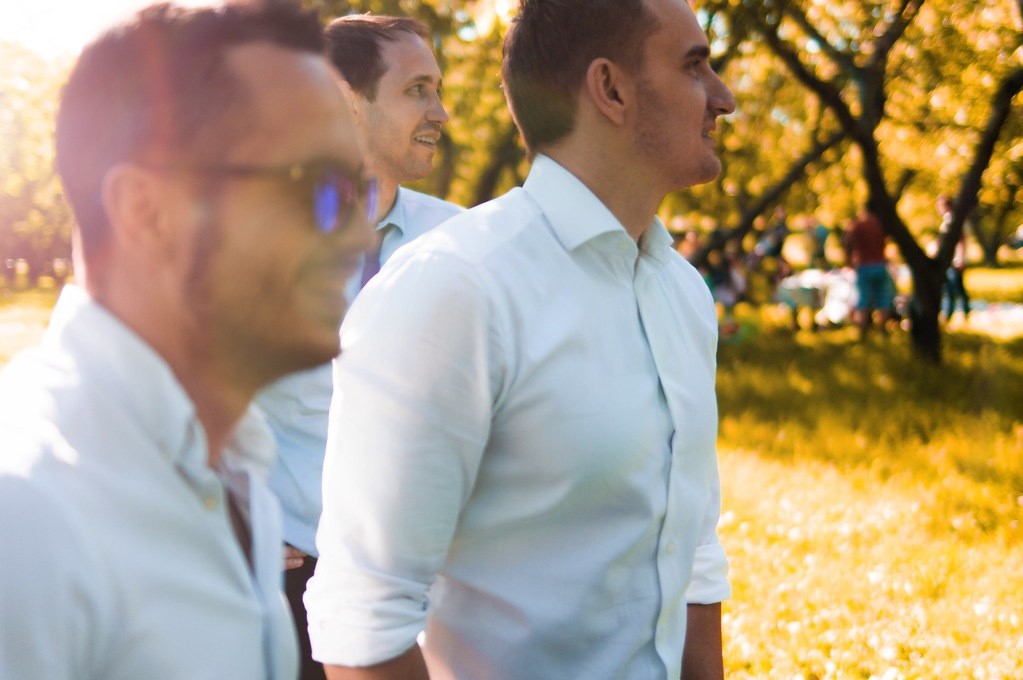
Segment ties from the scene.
[359,224,393,287]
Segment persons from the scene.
[682,195,971,343]
[0,0,376,680]
[302,0,736,680]
[242,14,472,680]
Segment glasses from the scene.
[149,154,379,236]
